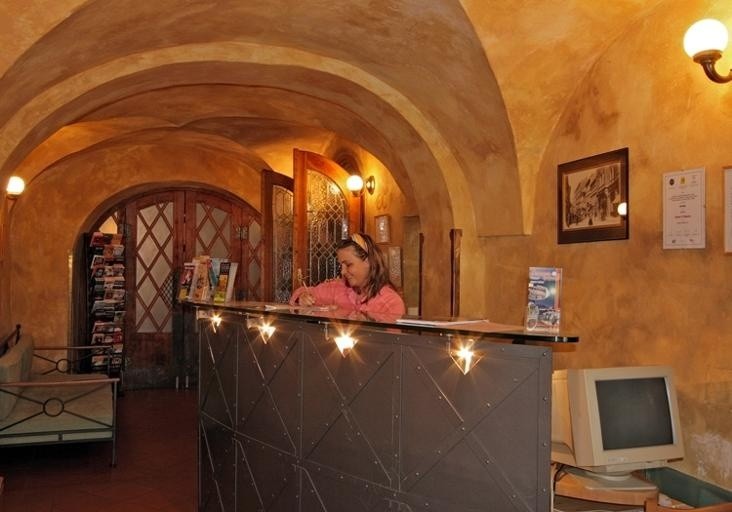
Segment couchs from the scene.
[2,321,123,469]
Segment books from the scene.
[90,230,127,372]
[175,253,238,303]
[523,264,563,335]
[396,317,484,327]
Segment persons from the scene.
[290,233,406,324]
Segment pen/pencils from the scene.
[302,281,309,292]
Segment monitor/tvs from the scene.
[549,365,685,490]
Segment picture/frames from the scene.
[554,147,631,246]
